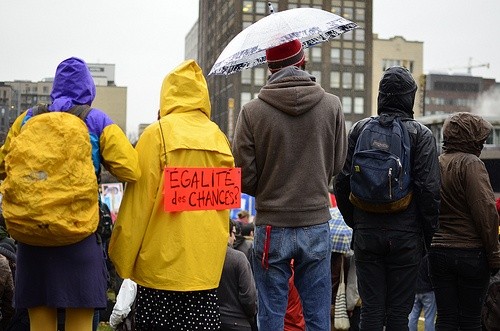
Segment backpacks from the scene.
[0,103,100,247]
[349,115,418,215]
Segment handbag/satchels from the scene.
[333,255,350,329]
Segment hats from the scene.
[266,39,305,73]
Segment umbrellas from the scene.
[206,2,360,76]
[327,207,355,258]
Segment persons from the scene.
[0,197,500,331]
[332,65,442,331]
[0,57,142,331]
[109,59,236,331]
[232,37,348,331]
[426,112,500,331]
